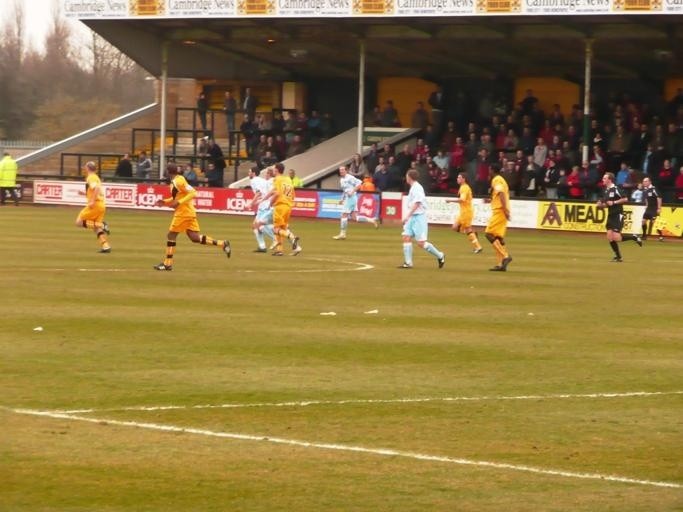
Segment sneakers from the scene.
[633,234,642,247]
[154,263,173,270]
[489,256,512,271]
[223,240,230,258]
[438,255,445,268]
[254,235,301,256]
[333,234,346,239]
[98,222,111,253]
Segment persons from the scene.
[0,149,19,206]
[166,86,332,188]
[446,172,483,254]
[641,177,663,240]
[332,165,379,240]
[243,162,302,256]
[113,153,133,178]
[484,161,513,271]
[349,80,682,206]
[398,169,446,269]
[596,172,642,261]
[75,162,111,253]
[134,151,153,178]
[154,164,231,270]
